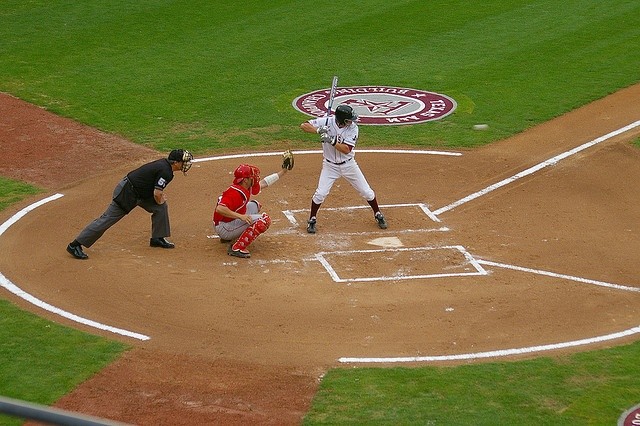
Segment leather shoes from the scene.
[150,237,175,248]
[66,243,88,259]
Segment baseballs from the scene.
[324,76,339,126]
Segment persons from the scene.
[211,150,294,259]
[65,149,193,259]
[300,104,389,235]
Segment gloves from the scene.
[316,125,330,134]
[321,133,337,146]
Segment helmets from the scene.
[168,149,193,176]
[233,165,260,195]
[336,105,357,126]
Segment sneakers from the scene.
[227,244,251,258]
[307,218,317,233]
[375,214,387,229]
[220,238,232,243]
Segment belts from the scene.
[326,158,352,164]
[123,176,141,201]
